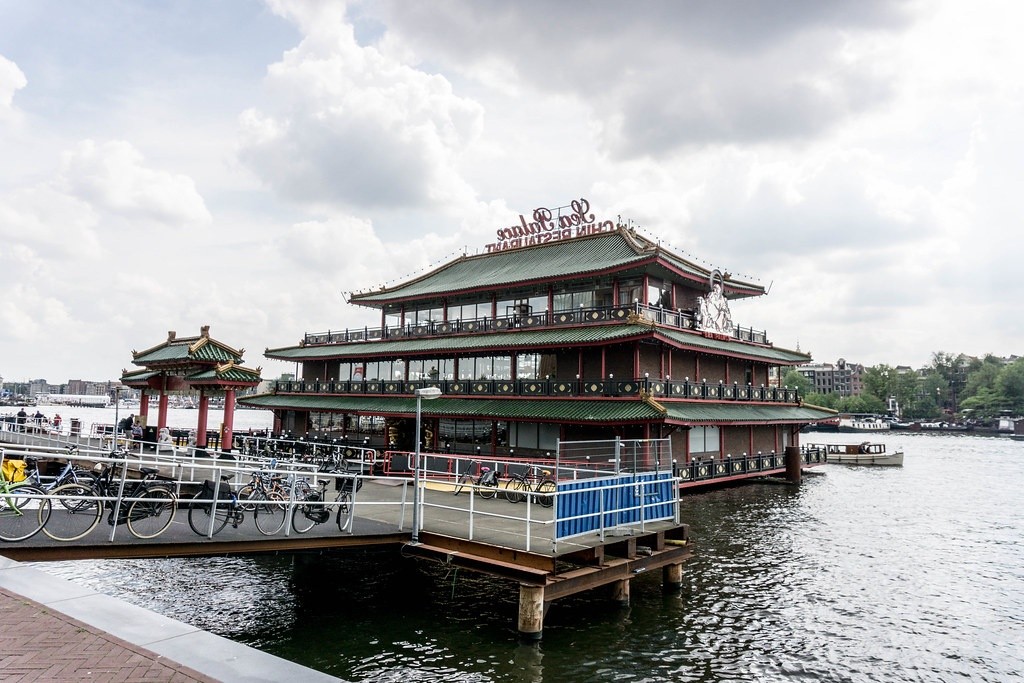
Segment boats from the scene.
[802,419,891,433]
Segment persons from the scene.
[866,446,870,453]
[18,408,26,430]
[125,414,143,451]
[35,411,43,426]
[54,414,62,429]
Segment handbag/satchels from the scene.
[202,479,230,508]
[2,459,27,483]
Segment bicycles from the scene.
[505,463,556,508]
[187,438,363,537]
[0,446,178,542]
[453,457,500,500]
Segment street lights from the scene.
[413,387,444,542]
[113,386,129,451]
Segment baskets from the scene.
[335,477,363,492]
